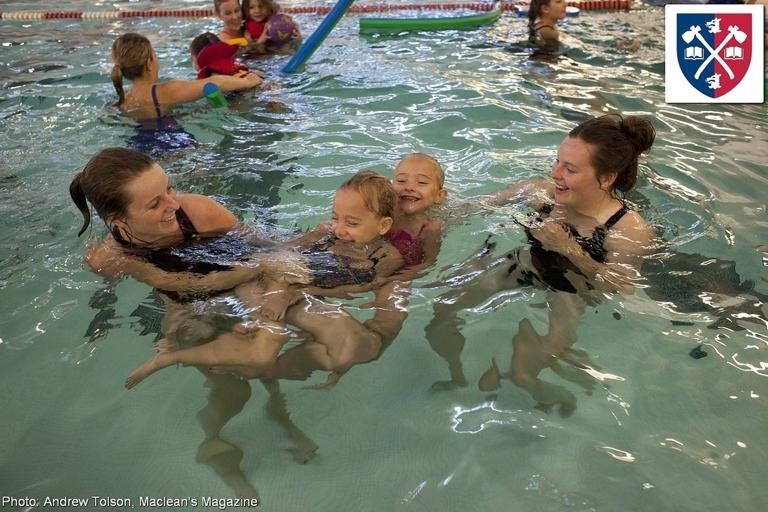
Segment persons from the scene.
[124,170,402,387]
[311,151,447,390]
[527,0,642,52]
[241,0,304,55]
[189,33,250,103]
[68,147,321,507]
[419,110,656,419]
[214,1,243,38]
[105,37,262,149]
